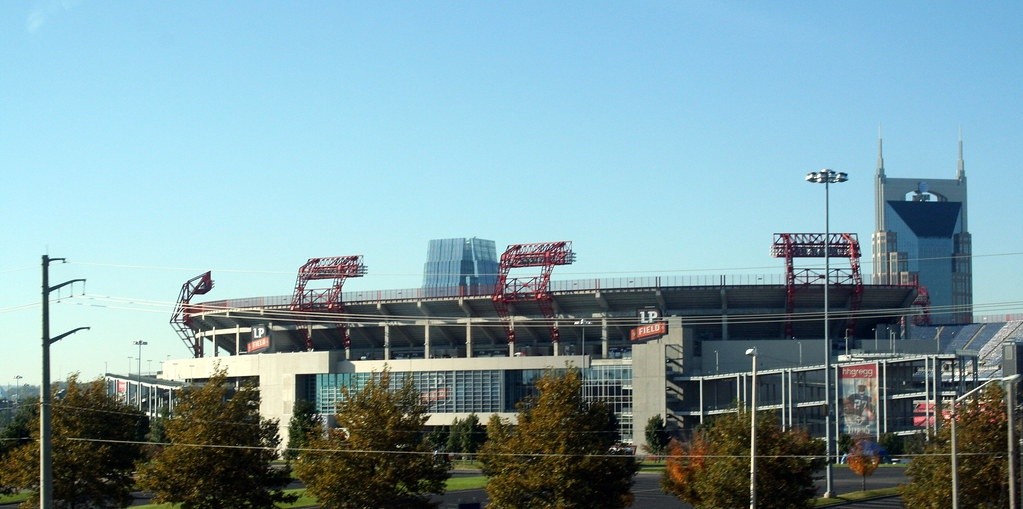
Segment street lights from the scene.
[14,374,23,407]
[935,326,941,353]
[886,327,893,353]
[745,347,759,509]
[573,318,593,410]
[792,337,803,366]
[844,328,854,355]
[872,328,878,352]
[891,332,896,354]
[134,340,148,412]
[804,168,849,497]
[713,349,719,374]
[949,373,1022,509]
